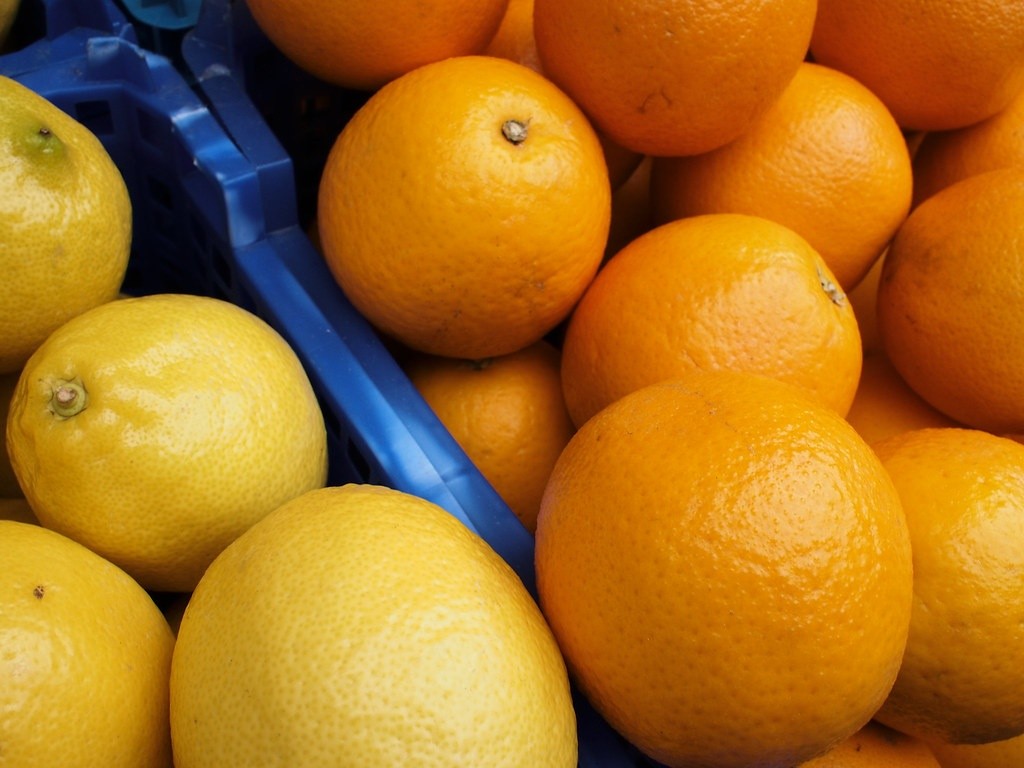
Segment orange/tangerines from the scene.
[256,0,1024,768]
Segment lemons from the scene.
[0,70,580,768]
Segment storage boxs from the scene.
[1,1,658,768]
